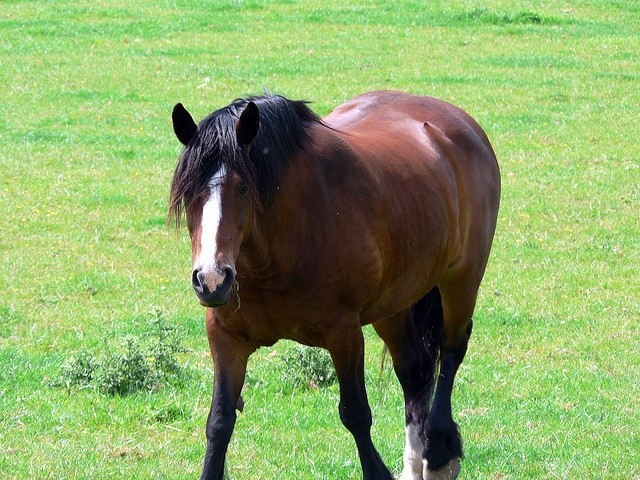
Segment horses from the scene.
[167,85,501,480]
